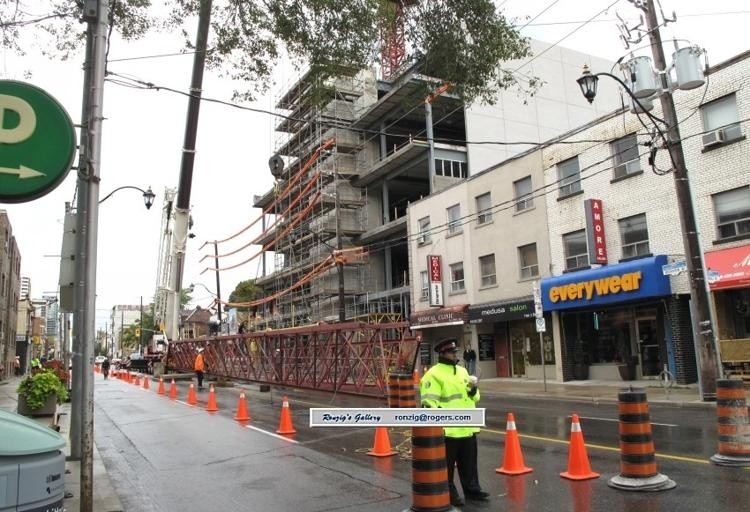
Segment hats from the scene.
[433,337,463,355]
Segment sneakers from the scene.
[446,492,464,508]
[465,489,492,502]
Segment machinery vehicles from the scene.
[142,0,211,374]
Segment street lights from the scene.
[575,65,674,174]
[189,282,217,298]
[577,64,718,400]
[97,182,157,210]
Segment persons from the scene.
[462,342,475,378]
[30,354,43,373]
[542,334,556,367]
[14,354,21,375]
[101,357,110,380]
[418,335,490,506]
[193,347,207,391]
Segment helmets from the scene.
[197,346,206,354]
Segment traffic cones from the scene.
[187,383,198,405]
[142,375,149,389]
[117,372,141,384]
[143,376,149,390]
[169,380,177,398]
[205,383,218,412]
[168,377,178,398]
[185,384,200,404]
[134,374,140,385]
[203,384,218,411]
[128,374,133,383]
